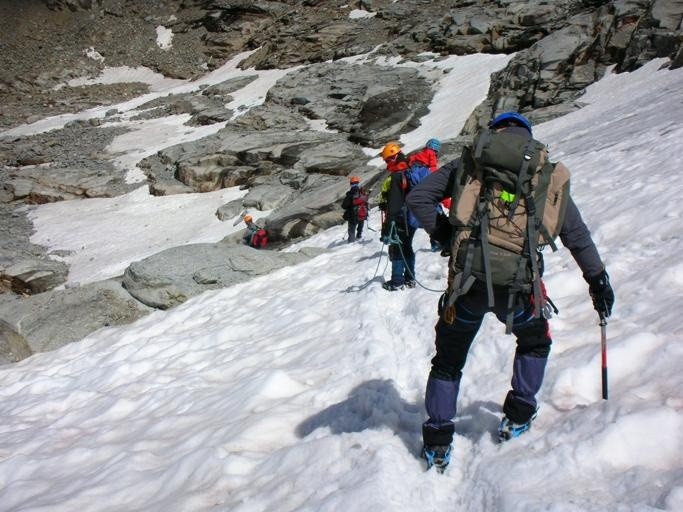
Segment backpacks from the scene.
[439,132,570,334]
[251,228,266,248]
[405,163,430,228]
[352,188,368,221]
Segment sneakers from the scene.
[383,278,415,291]
[496,412,537,442]
[421,445,451,473]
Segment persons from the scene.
[340,177,373,244]
[374,143,418,291]
[408,138,451,253]
[243,213,259,245]
[405,110,615,474]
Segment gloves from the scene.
[584,269,613,316]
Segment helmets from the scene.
[383,144,401,159]
[350,177,360,184]
[426,139,440,152]
[489,112,531,132]
[244,216,252,222]
